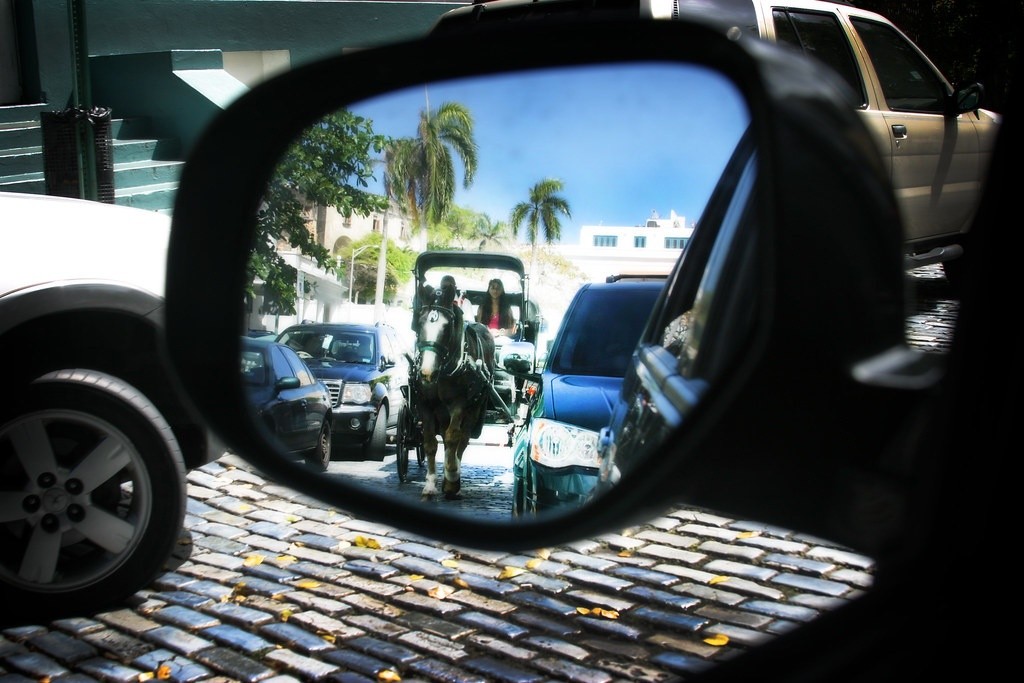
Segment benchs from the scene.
[424,288,521,337]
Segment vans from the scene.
[433,0,999,285]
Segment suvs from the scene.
[272,321,409,461]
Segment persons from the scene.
[435,275,475,323]
[477,279,516,336]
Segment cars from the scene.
[0,190,228,618]
[241,341,336,472]
[503,274,670,517]
[874,379,885,387]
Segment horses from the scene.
[411,279,496,503]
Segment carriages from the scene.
[395,250,539,498]
